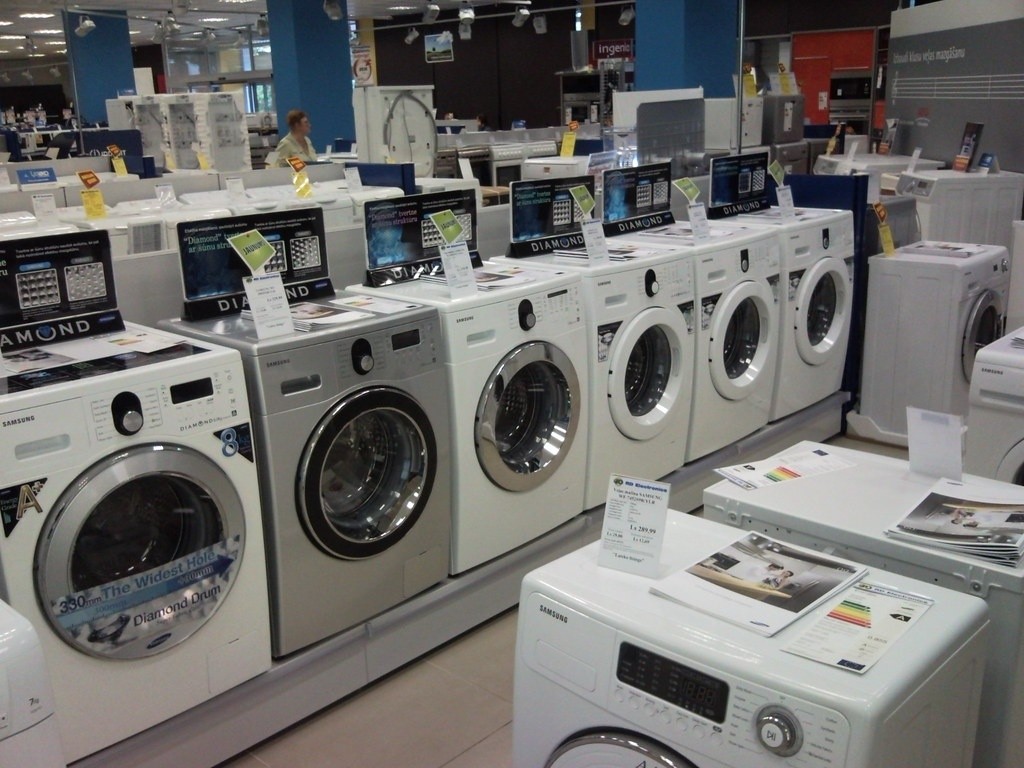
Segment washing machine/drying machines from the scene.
[722,204,854,424]
[489,233,697,514]
[513,506,991,767]
[702,440,1024,765]
[961,324,1024,486]
[863,239,1013,431]
[611,219,779,465]
[159,286,456,664]
[1,319,275,768]
[346,257,592,576]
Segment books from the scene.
[883,477,1024,567]
[639,226,732,239]
[241,302,374,332]
[553,246,637,261]
[739,209,806,219]
[422,270,514,288]
[649,531,870,638]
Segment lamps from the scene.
[151,0,191,44]
[0,71,9,83]
[255,13,268,36]
[533,13,547,34]
[323,0,344,20]
[422,0,440,24]
[458,23,471,40]
[22,36,37,55]
[458,0,475,24]
[21,69,31,81]
[511,5,530,28]
[404,27,419,45]
[48,66,60,78]
[348,32,360,45]
[197,29,215,47]
[618,5,635,25]
[232,31,246,47]
[74,15,95,37]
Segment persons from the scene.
[744,563,785,579]
[438,109,460,134]
[476,113,492,130]
[764,570,793,589]
[951,509,975,524]
[963,512,983,527]
[841,122,861,135]
[263,108,318,169]
